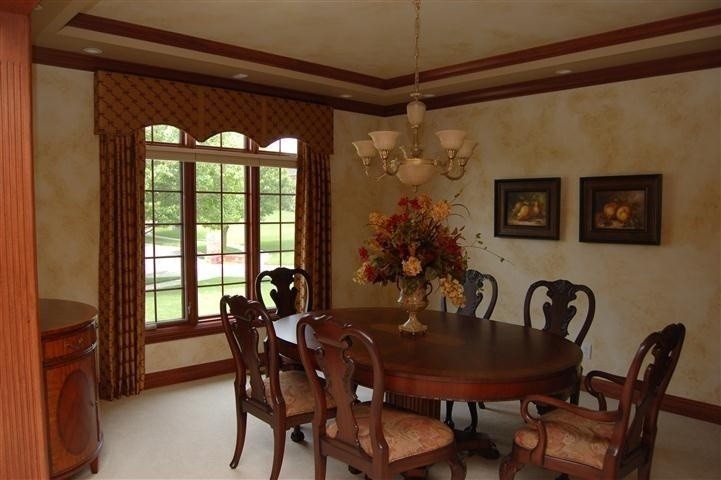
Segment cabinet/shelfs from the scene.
[39,298,104,480]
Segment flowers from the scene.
[352,174,514,308]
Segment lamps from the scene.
[351,0,478,197]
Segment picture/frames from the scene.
[580,174,662,244]
[495,178,560,240]
